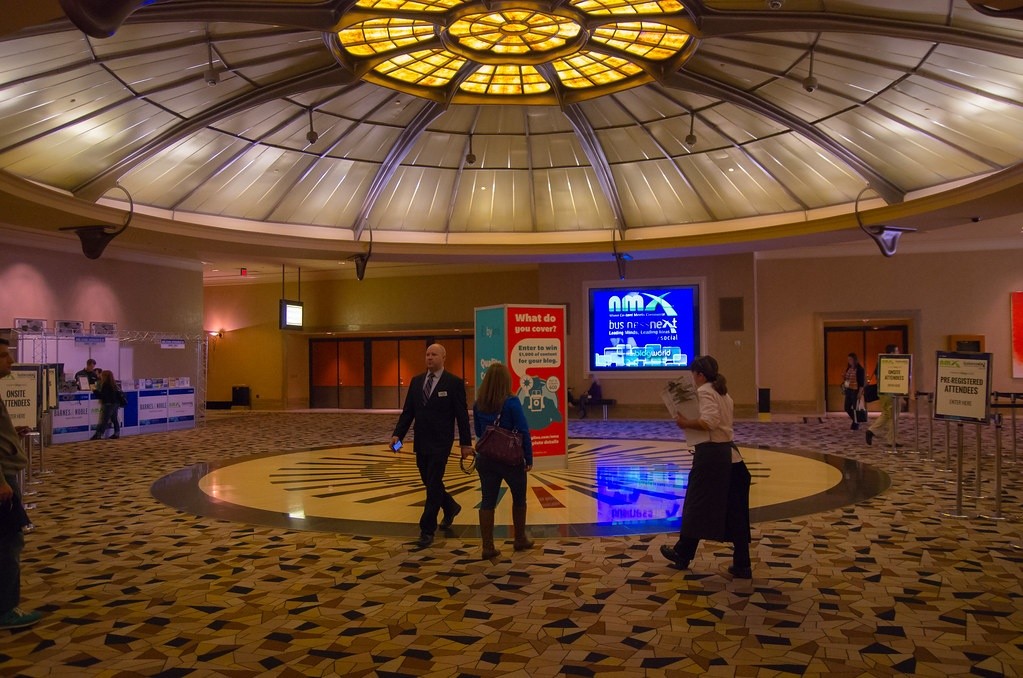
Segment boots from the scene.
[478,509,501,559]
[512,503,535,549]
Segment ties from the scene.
[422,373,435,406]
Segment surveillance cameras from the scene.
[306,131,318,144]
[466,154,476,165]
[803,77,819,93]
[204,71,219,87]
[685,135,696,148]
[769,0,782,10]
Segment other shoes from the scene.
[90,434,102,440]
[887,442,903,447]
[728,565,752,580]
[851,421,860,430]
[866,430,872,445]
[660,545,690,569]
[109,435,119,439]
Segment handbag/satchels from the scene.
[863,384,879,402]
[115,384,128,406]
[475,394,524,466]
[856,399,867,422]
[854,396,866,424]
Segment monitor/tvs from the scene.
[589,285,699,371]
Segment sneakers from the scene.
[417,533,434,546]
[439,505,461,529]
[0,606,43,630]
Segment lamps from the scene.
[0,0,1023,283]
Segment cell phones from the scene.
[392,439,403,453]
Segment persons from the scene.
[568,374,602,419]
[0,337,44,629]
[75,358,98,385]
[474,362,535,560]
[387,344,473,549]
[840,352,865,430]
[94,368,103,388]
[858,343,906,448]
[89,370,121,440]
[659,354,753,579]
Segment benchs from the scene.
[585,398,616,420]
